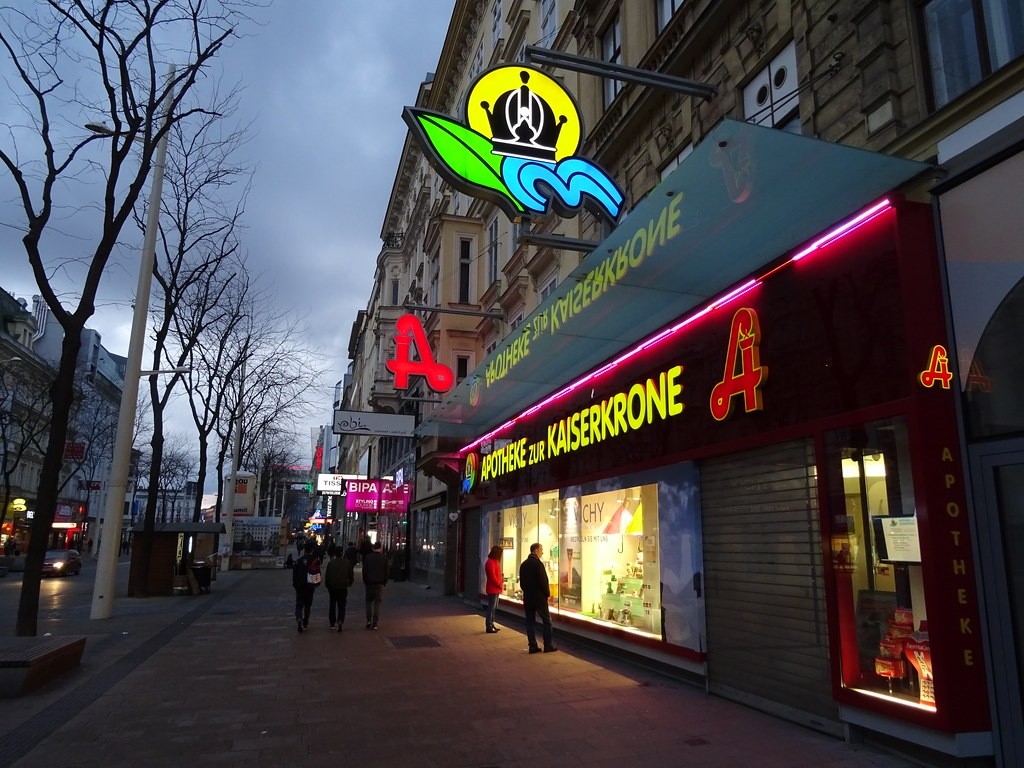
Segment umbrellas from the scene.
[626,503,643,552]
[603,505,632,553]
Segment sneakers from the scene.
[373,624,378,629]
[544,646,559,653]
[528,647,542,653]
[330,621,336,630]
[366,621,371,627]
[337,624,344,632]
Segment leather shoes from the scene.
[486,628,498,633]
[490,625,501,631]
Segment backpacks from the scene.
[362,553,386,585]
[307,560,321,588]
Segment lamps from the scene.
[549,500,558,518]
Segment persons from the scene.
[68,538,83,550]
[4,539,16,556]
[519,543,557,654]
[362,541,388,630]
[325,547,354,632]
[123,539,127,553]
[361,536,372,559]
[293,535,343,564]
[88,538,93,552]
[293,544,322,633]
[485,546,503,633]
[345,543,357,566]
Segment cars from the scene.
[43,548,82,580]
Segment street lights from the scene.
[207,374,244,573]
[82,122,194,622]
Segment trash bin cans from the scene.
[189,566,212,595]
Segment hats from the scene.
[343,560,354,570]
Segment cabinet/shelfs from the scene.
[588,558,644,620]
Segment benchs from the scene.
[1,634,87,693]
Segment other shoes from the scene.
[297,619,303,632]
[303,622,308,628]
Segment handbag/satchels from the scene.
[331,580,347,599]
